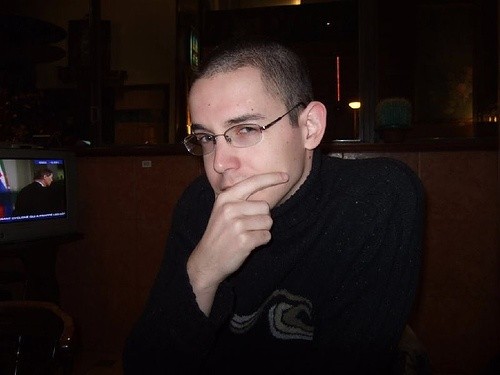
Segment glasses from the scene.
[181,101,307,156]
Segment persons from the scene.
[121,32,428,375]
[14,168,54,217]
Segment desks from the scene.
[0,232,85,301]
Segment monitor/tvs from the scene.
[0,148,77,244]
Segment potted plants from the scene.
[375,99,412,143]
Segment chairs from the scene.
[0,301,81,375]
